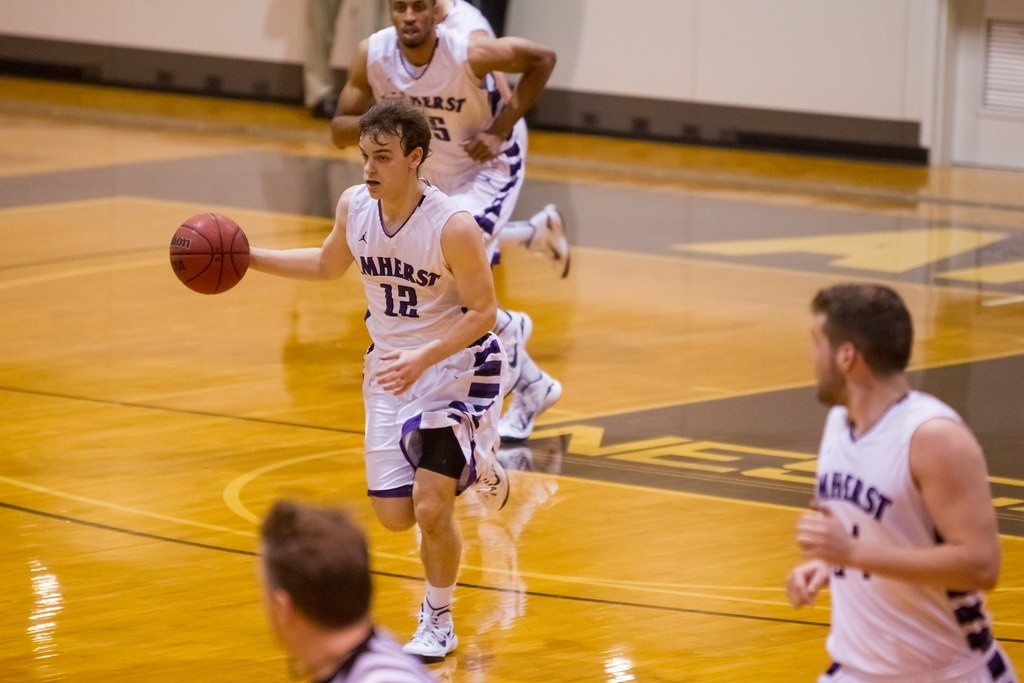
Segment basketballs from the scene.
[169,213,250,295]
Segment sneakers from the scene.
[403,603,457,663]
[472,426,510,511]
[495,310,533,399]
[525,204,570,278]
[497,370,561,444]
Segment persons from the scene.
[251,0,572,658]
[785,279,1023,683]
[258,499,443,683]
